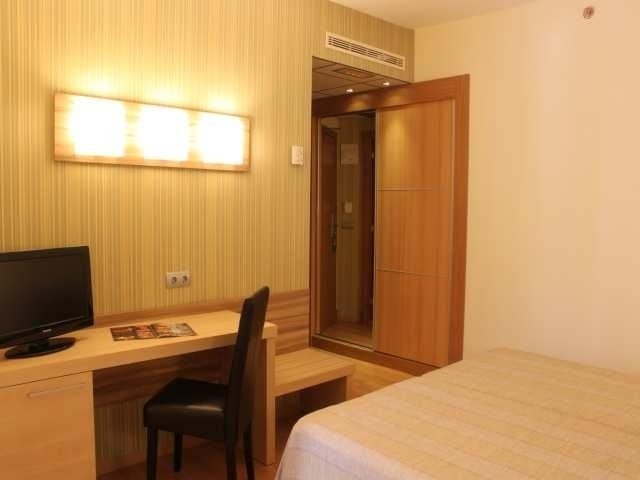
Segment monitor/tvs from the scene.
[0,246,94,359]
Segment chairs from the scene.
[141,285,270,480]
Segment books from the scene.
[109,321,198,340]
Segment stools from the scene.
[275,347,359,405]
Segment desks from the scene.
[1,310,278,480]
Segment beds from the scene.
[280,347,639,480]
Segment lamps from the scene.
[52,88,254,173]
